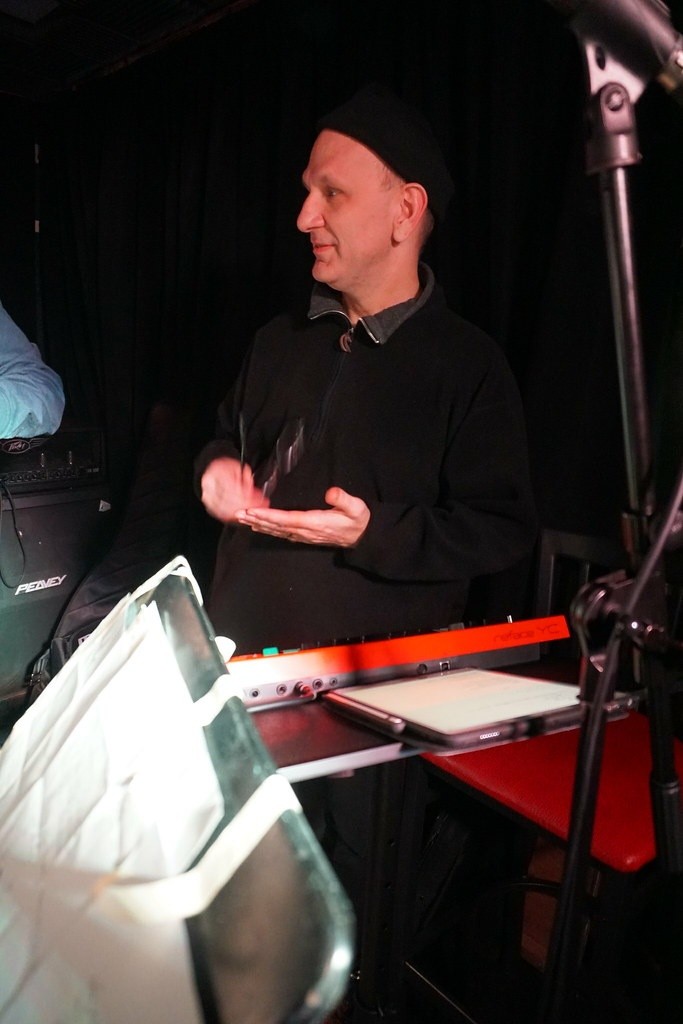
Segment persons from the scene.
[191,91,541,1023]
[0,296,66,441]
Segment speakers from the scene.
[0,486,120,716]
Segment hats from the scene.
[314,81,457,229]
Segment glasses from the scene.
[236,406,308,507]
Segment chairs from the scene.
[389,530,683,1024]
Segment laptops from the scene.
[330,668,641,747]
